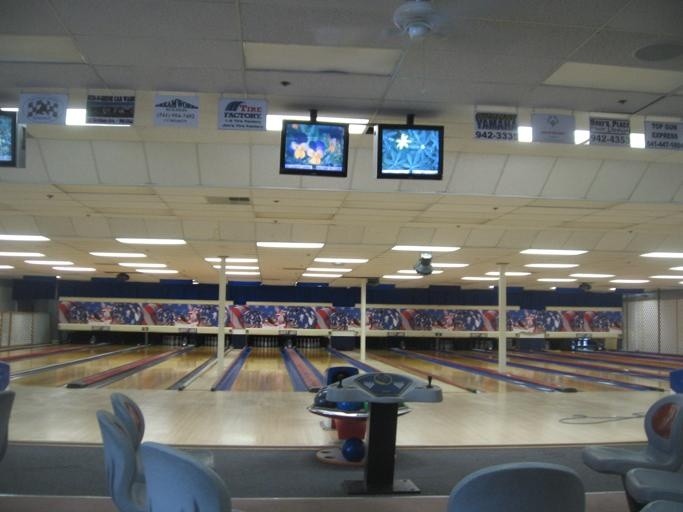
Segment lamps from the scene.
[415,254,433,277]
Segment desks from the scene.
[296,361,443,500]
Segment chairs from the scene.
[140,441,233,511]
[96,410,147,511]
[581,393,683,476]
[624,465,683,511]
[111,392,217,487]
[448,461,587,512]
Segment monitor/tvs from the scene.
[0,105,29,168]
[279,118,350,179]
[371,122,444,182]
